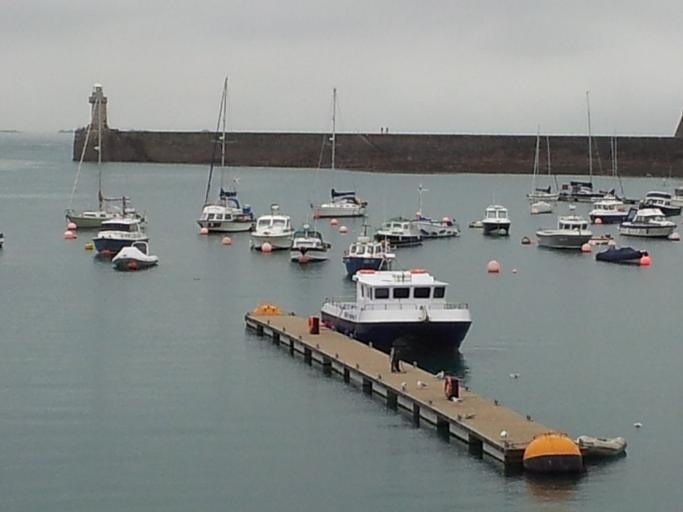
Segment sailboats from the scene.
[526,91,642,215]
[196,77,259,233]
[310,86,368,217]
[64,87,146,228]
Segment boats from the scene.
[534,205,594,250]
[638,187,683,218]
[589,194,632,223]
[595,239,651,265]
[341,223,397,277]
[618,207,677,238]
[92,208,151,256]
[289,223,332,264]
[469,220,483,229]
[592,233,614,245]
[318,267,469,352]
[373,185,463,248]
[480,204,512,236]
[250,204,296,253]
[111,240,159,271]
